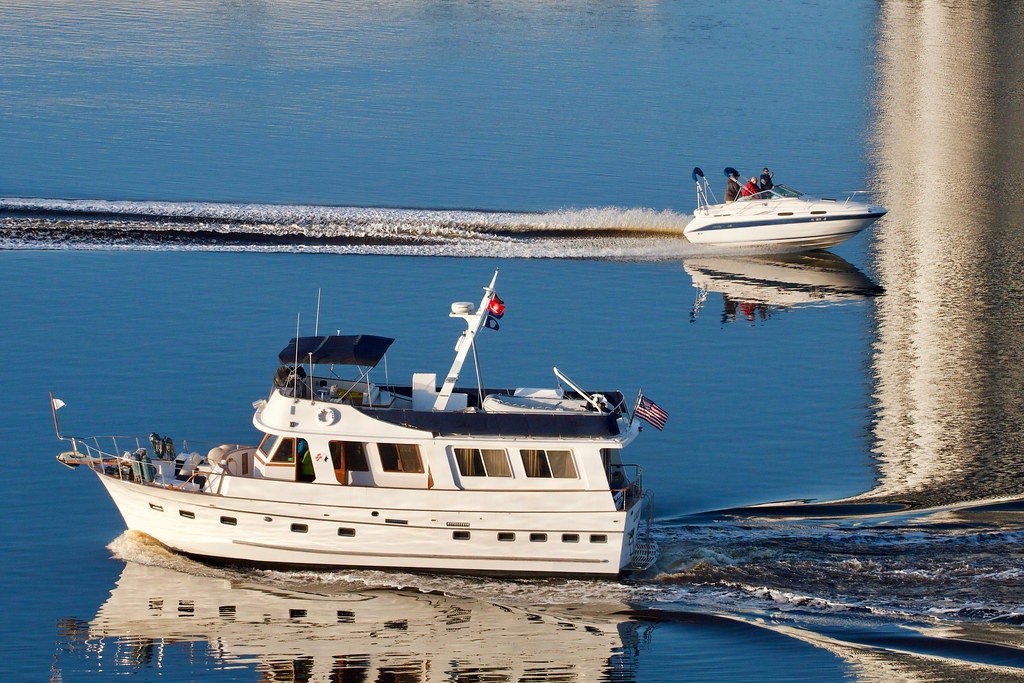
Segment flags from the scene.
[485,294,504,332]
[633,395,669,431]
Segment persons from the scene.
[760,168,774,199]
[742,177,761,199]
[725,173,742,205]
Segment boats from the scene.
[684,168,885,247]
[684,255,885,310]
[74,562,638,682]
[52,268,660,580]
